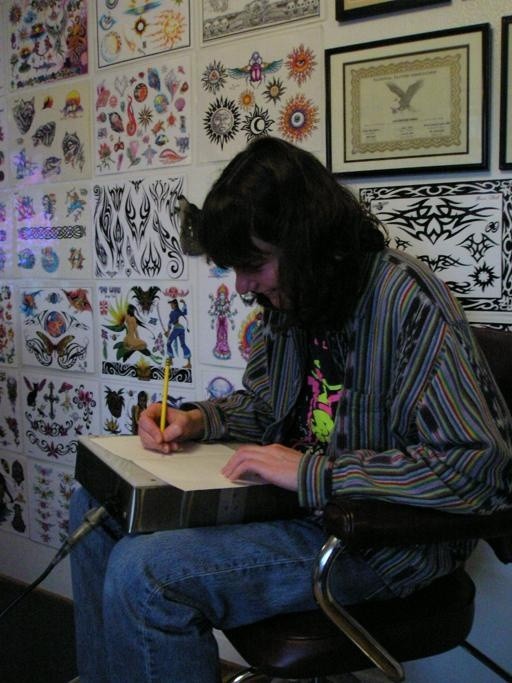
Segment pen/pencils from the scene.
[160,357,171,454]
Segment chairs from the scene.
[222,329,512,682]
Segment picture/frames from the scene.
[323,23,489,180]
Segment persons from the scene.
[133,390,148,434]
[209,283,239,360]
[164,298,193,368]
[66,134,512,683]
